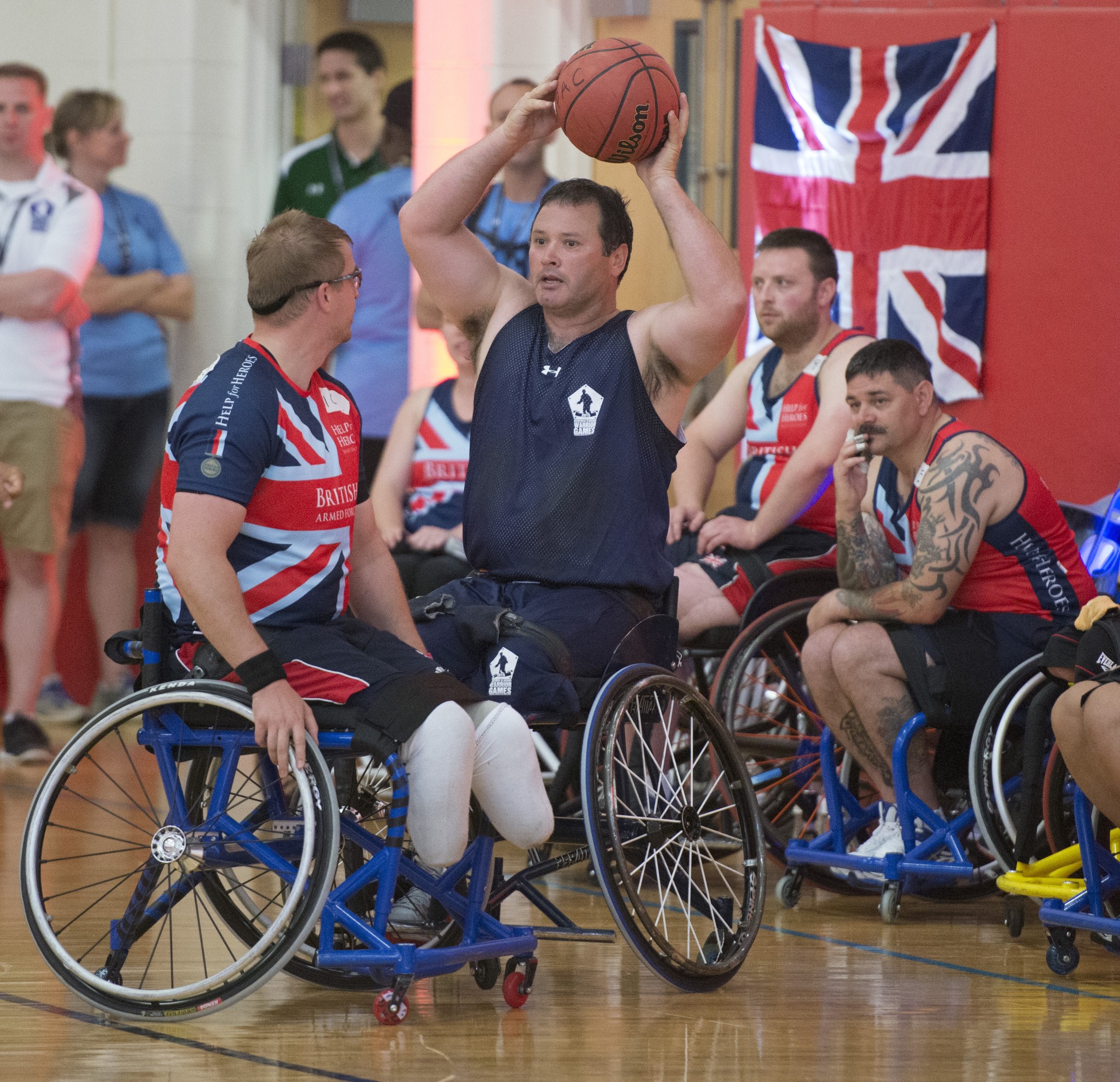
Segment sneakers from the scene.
[385,885,447,940]
[830,800,971,885]
[93,672,143,721]
[1,713,55,765]
[35,680,90,725]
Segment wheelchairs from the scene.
[437,497,1120,997]
[18,580,541,1029]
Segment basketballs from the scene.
[555,37,680,164]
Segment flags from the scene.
[756,20,997,406]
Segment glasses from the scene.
[329,268,363,289]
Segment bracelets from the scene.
[235,646,286,694]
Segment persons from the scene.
[269,27,411,504]
[0,63,198,761]
[153,208,557,864]
[369,77,1120,942]
